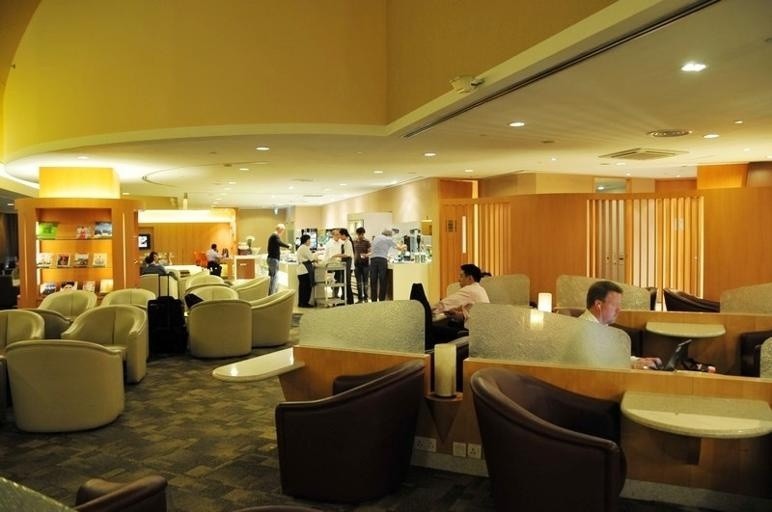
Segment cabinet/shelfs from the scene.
[36,236,113,302]
[313,263,346,307]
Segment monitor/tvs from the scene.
[139,234,150,249]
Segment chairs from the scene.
[73,475,172,511]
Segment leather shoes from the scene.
[301,304,313,307]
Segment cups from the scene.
[414,252,426,264]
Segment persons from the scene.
[431,262,491,343]
[267,223,292,295]
[141,253,178,279]
[207,242,223,277]
[353,227,373,304]
[323,229,345,299]
[557,279,663,372]
[296,235,320,308]
[329,229,356,306]
[142,252,159,266]
[371,229,407,303]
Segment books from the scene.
[36,251,51,268]
[94,221,113,237]
[75,225,91,239]
[82,280,96,293]
[60,280,78,292]
[75,252,89,266]
[40,281,56,298]
[93,252,107,266]
[100,279,114,294]
[57,254,71,267]
[37,221,59,239]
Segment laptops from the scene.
[650,338,692,371]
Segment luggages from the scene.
[148,274,188,362]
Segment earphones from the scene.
[597,303,603,312]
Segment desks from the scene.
[621,394,771,465]
[646,321,726,340]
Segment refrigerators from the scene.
[345,210,392,298]
